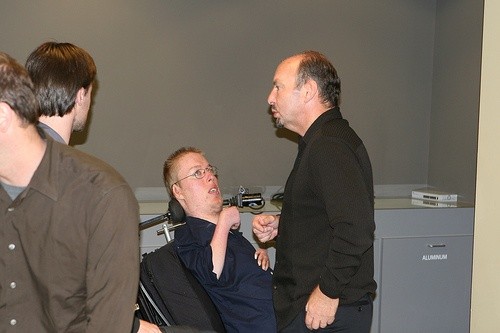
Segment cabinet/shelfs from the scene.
[137,196,476,333]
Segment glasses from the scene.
[169,166,217,188]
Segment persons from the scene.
[164,147,279,333]
[0,52,140,333]
[253,50,377,333]
[25,41,162,333]
